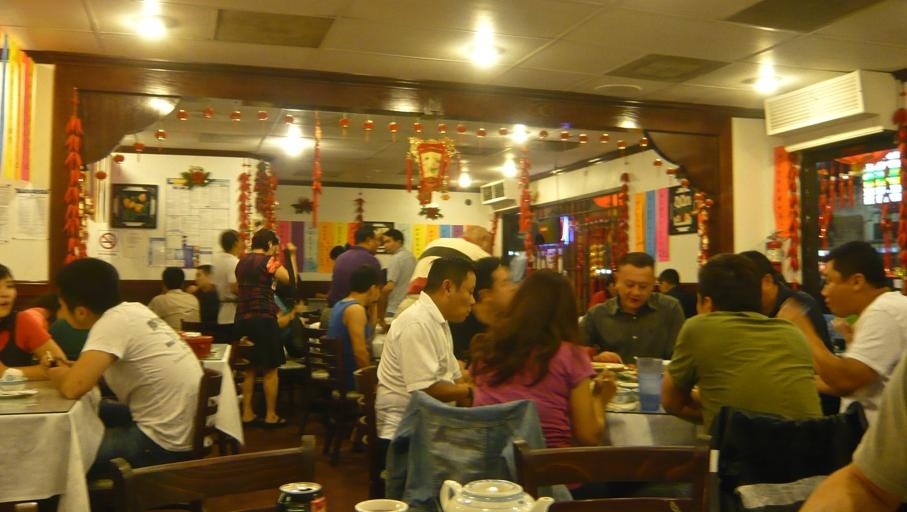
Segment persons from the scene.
[1,254,204,480]
[373,243,907,511]
[317,221,490,397]
[149,231,302,430]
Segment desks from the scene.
[568,345,701,447]
[0,364,102,512]
[369,315,399,357]
[171,338,239,456]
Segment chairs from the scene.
[301,325,328,371]
[379,389,544,506]
[193,367,222,457]
[512,436,719,507]
[353,364,390,497]
[716,402,868,497]
[304,339,364,461]
[279,360,308,410]
[112,433,320,509]
[224,336,255,454]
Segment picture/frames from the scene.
[668,183,697,235]
[110,182,159,229]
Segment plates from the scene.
[589,362,624,370]
[0,390,39,397]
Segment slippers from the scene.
[243,415,265,426]
[263,417,289,426]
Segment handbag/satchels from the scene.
[279,316,311,360]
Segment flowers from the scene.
[290,195,320,214]
[418,207,444,219]
[182,166,217,190]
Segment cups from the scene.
[638,357,662,411]
[610,387,631,404]
[355,498,407,511]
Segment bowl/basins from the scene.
[0,378,28,394]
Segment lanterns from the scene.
[93,101,715,211]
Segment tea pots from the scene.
[438,477,554,512]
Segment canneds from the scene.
[276,479,328,512]
